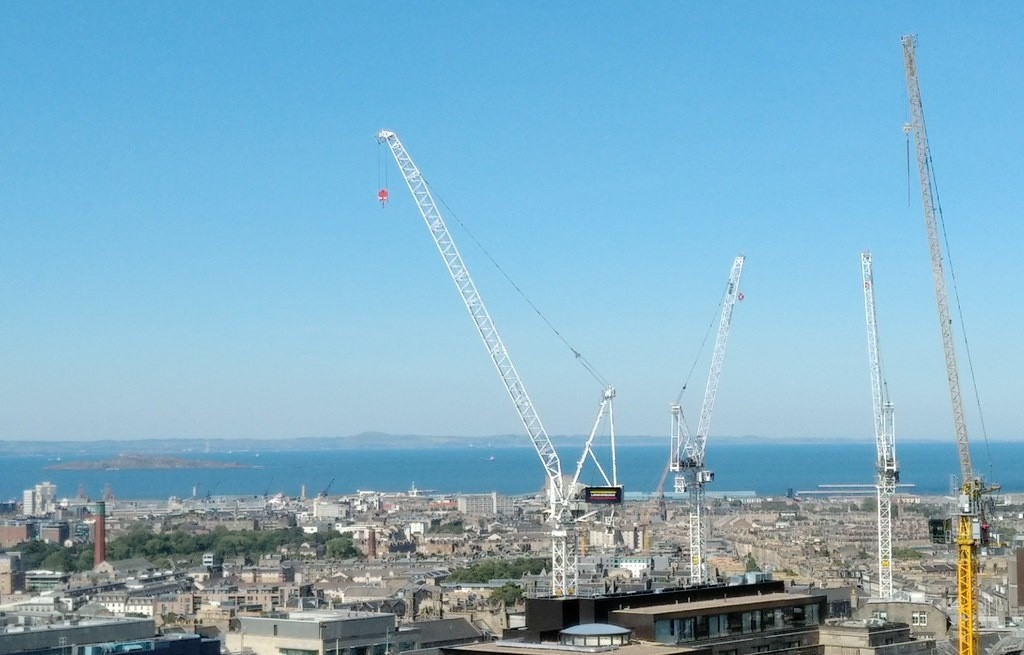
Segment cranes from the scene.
[376,28,1001,655]
